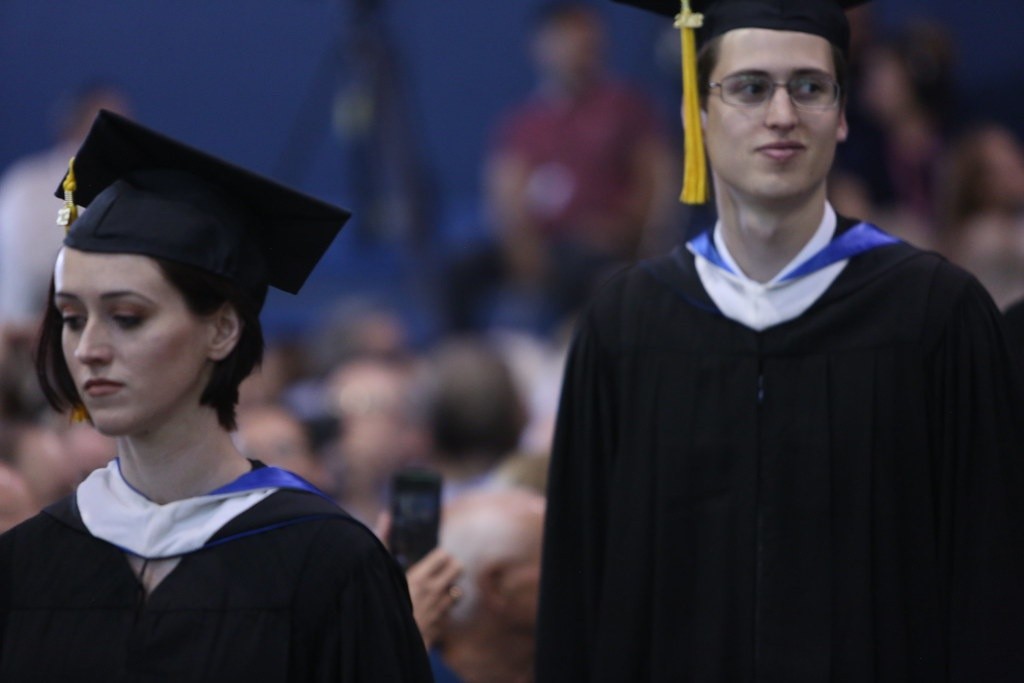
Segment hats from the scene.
[620,0,870,205]
[54,107,351,427]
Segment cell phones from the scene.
[388,468,441,572]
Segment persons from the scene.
[535,1,1024,682]
[0,79,568,683]
[473,3,1022,336]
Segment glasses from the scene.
[705,73,845,115]
